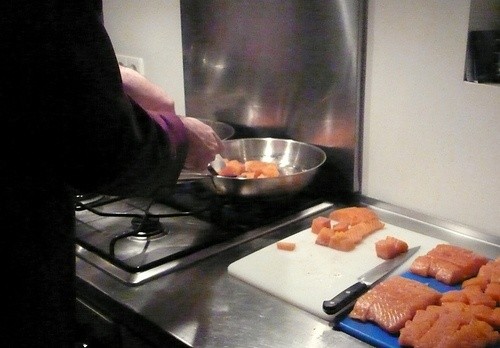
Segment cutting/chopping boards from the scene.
[335,271,463,348]
[226,221,450,321]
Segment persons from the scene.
[1,1,227,348]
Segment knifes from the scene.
[322,246,422,314]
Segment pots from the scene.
[176,138,328,198]
[200,119,235,141]
